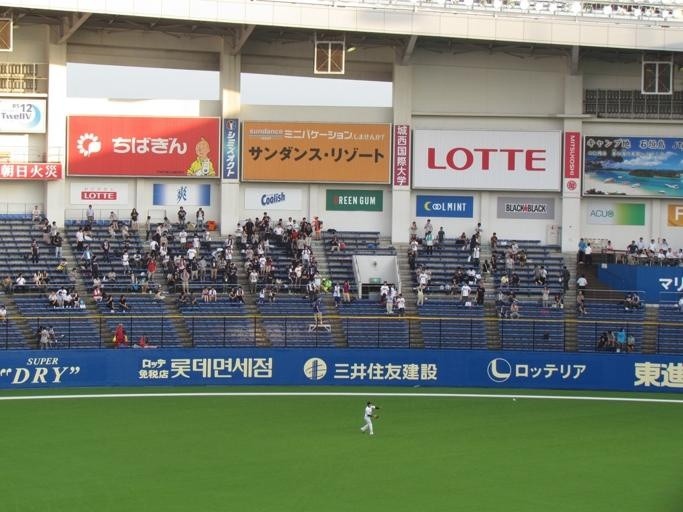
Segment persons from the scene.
[360,401,380,435]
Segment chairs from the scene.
[656,303,683,354]
[493,238,565,352]
[149,217,335,349]
[323,230,410,349]
[577,250,683,267]
[409,238,488,350]
[575,300,645,355]
[0,218,180,350]
